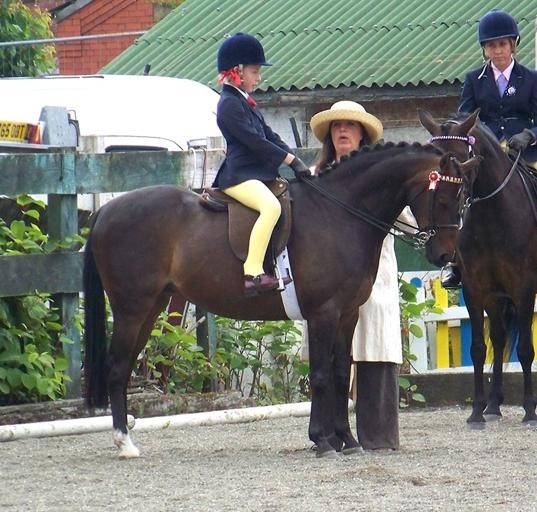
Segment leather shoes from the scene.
[244,275,292,296]
[441,263,459,286]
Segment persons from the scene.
[211,32,311,300]
[440,10,537,286]
[311,98,418,450]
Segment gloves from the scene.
[288,157,311,180]
[506,128,536,151]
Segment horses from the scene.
[81,141,485,461]
[415,105,537,431]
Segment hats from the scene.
[309,100,384,145]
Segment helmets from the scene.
[478,12,518,42]
[218,33,273,71]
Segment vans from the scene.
[0,73,306,392]
